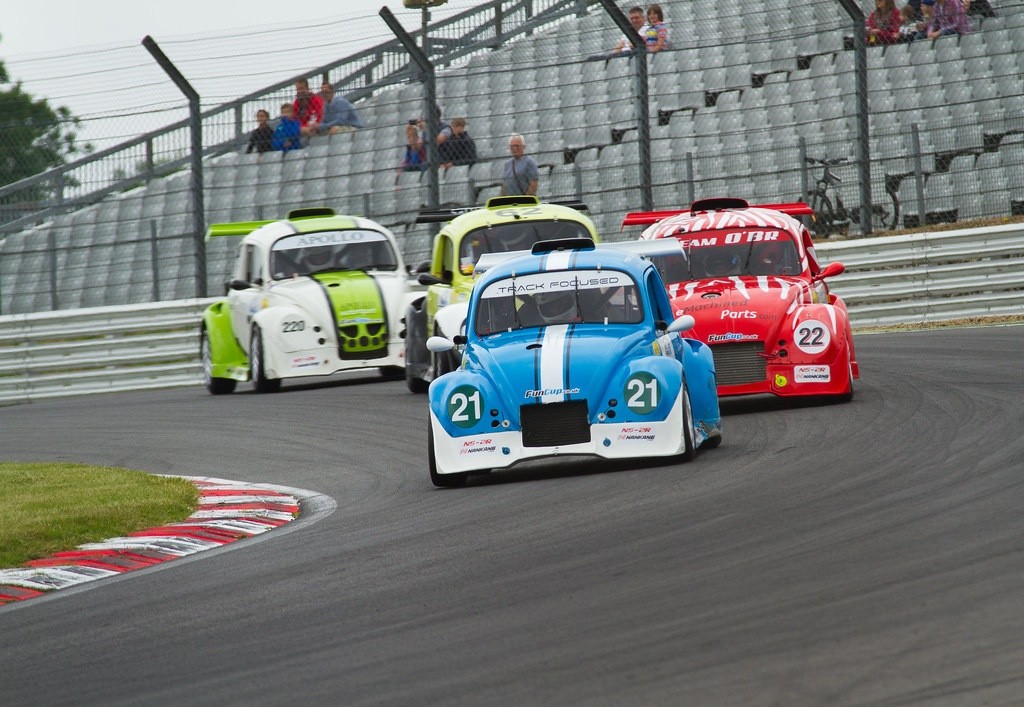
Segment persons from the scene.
[701,247,743,280]
[614,5,650,56]
[292,78,324,137]
[301,246,336,273]
[246,110,274,151]
[644,4,670,54]
[534,290,583,323]
[311,83,368,135]
[271,103,302,151]
[401,105,478,175]
[861,0,994,48]
[503,133,539,198]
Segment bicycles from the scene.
[794,155,899,240]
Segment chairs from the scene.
[0,2,1024,314]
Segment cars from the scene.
[618,196,861,402]
[195,206,433,397]
[423,238,722,488]
[401,194,603,399]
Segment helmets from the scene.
[535,291,578,324]
[498,228,530,252]
[702,247,741,278]
[306,246,335,273]
